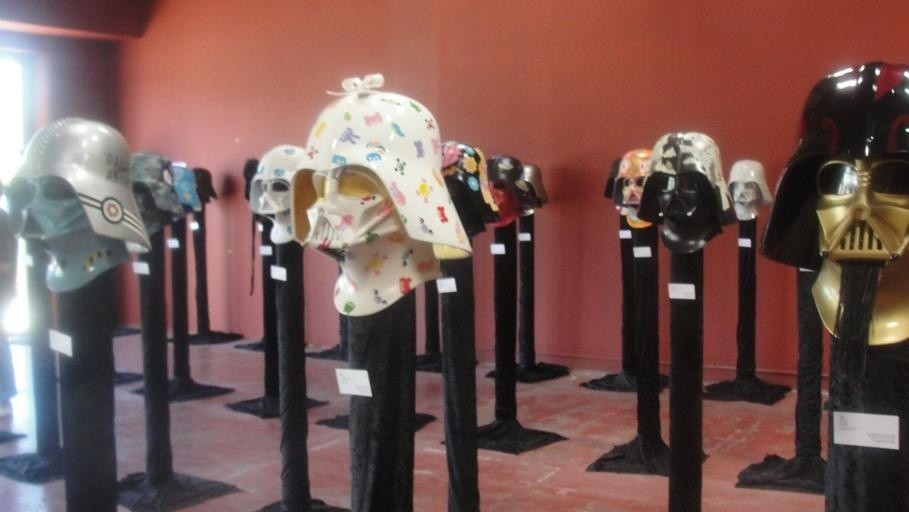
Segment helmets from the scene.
[6,117,217,251]
[758,61,907,271]
[602,132,774,226]
